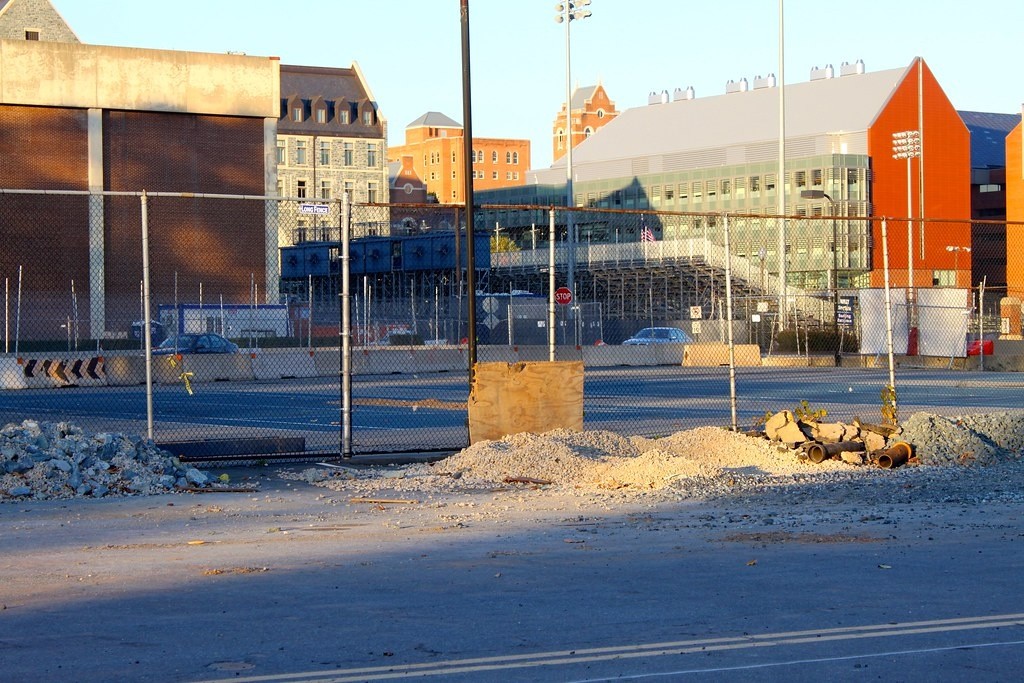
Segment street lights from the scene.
[801,190,841,367]
[557,0,590,320]
[892,131,920,323]
[738,253,759,311]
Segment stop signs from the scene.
[555,287,572,305]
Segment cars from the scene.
[622,328,693,345]
[151,332,239,354]
